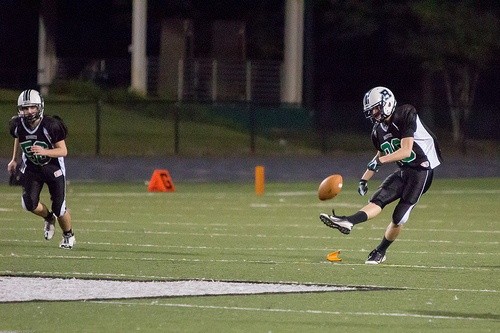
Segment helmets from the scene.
[363,87,397,125]
[18,89,44,124]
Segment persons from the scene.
[319,86,441,265]
[8,89,75,250]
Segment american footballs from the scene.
[318,173,343,201]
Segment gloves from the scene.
[367,157,383,173]
[357,179,369,197]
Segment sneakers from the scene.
[364,248,387,264]
[59,232,75,250]
[43,211,57,240]
[319,208,354,236]
